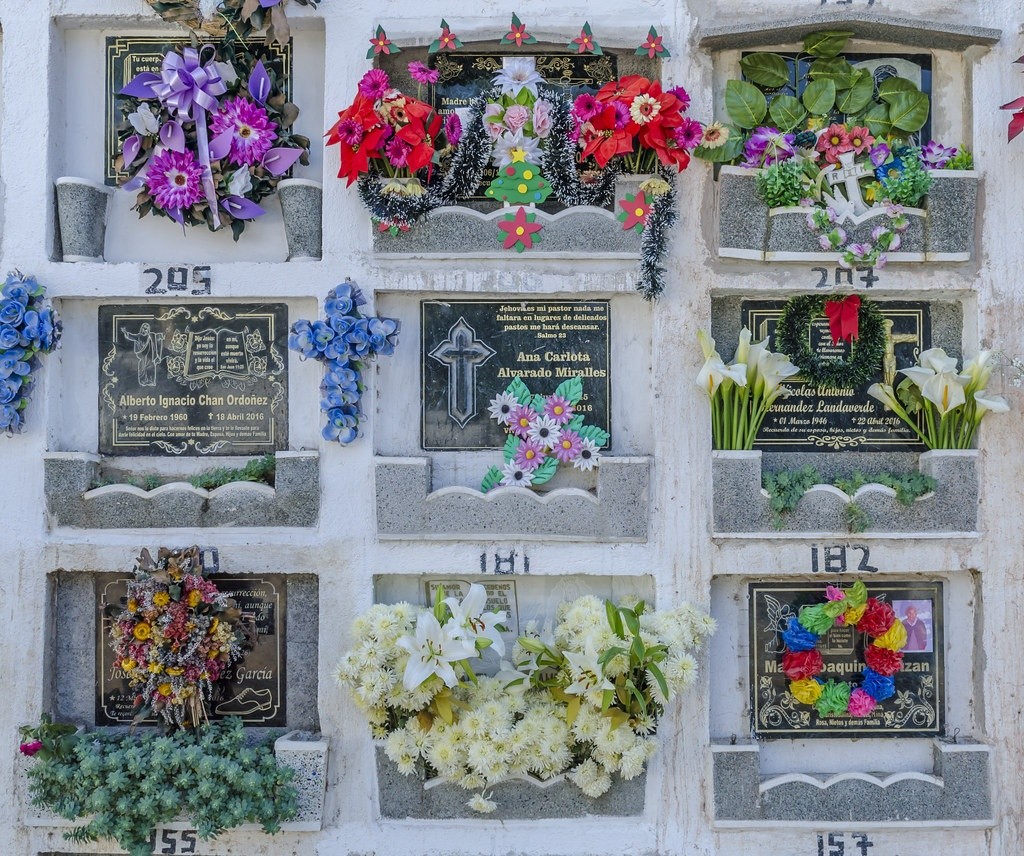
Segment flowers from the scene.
[332,583,718,813]
[111,45,310,233]
[287,277,400,446]
[867,348,1010,450]
[482,58,553,174]
[19,714,77,764]
[697,324,800,450]
[109,545,246,738]
[0,269,62,438]
[324,60,462,230]
[694,31,974,268]
[481,376,609,493]
[569,73,729,196]
